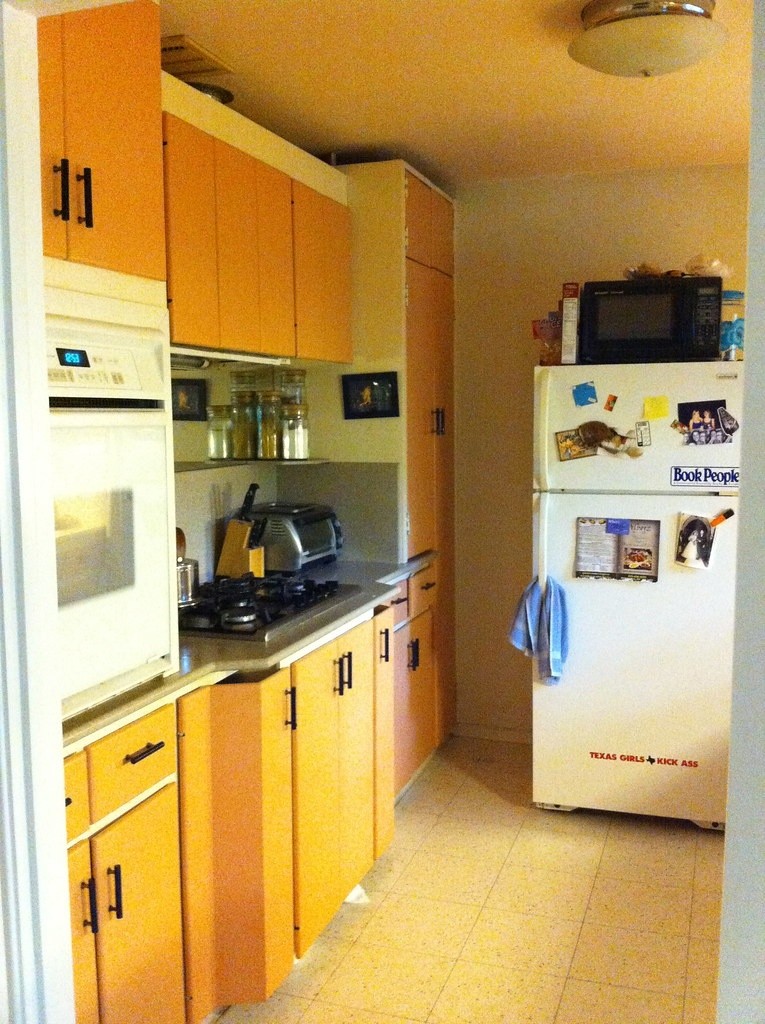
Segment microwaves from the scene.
[577,275,722,362]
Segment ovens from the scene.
[45,287,178,724]
[244,500,345,573]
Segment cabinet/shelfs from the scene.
[40,2,455,1024]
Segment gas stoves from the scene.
[178,570,361,640]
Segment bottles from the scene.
[205,368,310,460]
[721,289,745,360]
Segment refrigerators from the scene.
[529,360,744,830]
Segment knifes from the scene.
[236,483,268,549]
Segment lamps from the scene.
[566,0,721,78]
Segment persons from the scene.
[697,529,705,559]
[690,410,706,444]
[361,386,371,406]
[682,530,698,559]
[702,409,716,444]
